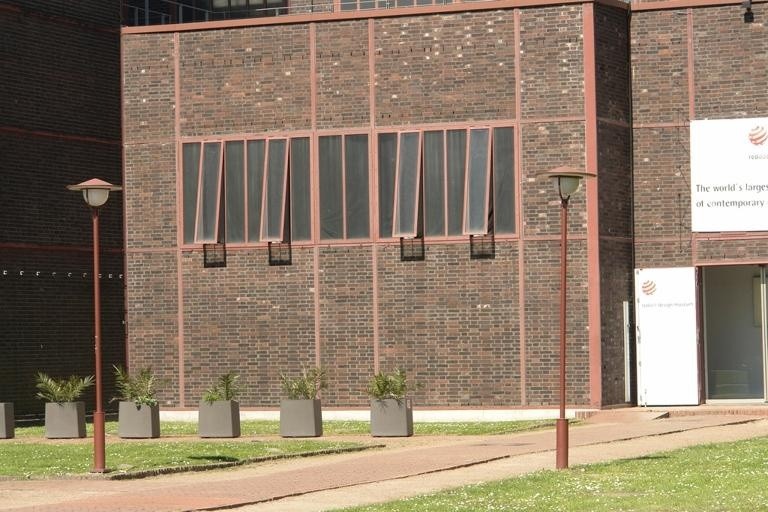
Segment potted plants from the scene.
[362,363,426,440]
[108,361,163,440]
[198,369,244,439]
[33,367,98,440]
[277,366,330,440]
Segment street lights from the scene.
[534,163,597,471]
[65,178,122,474]
